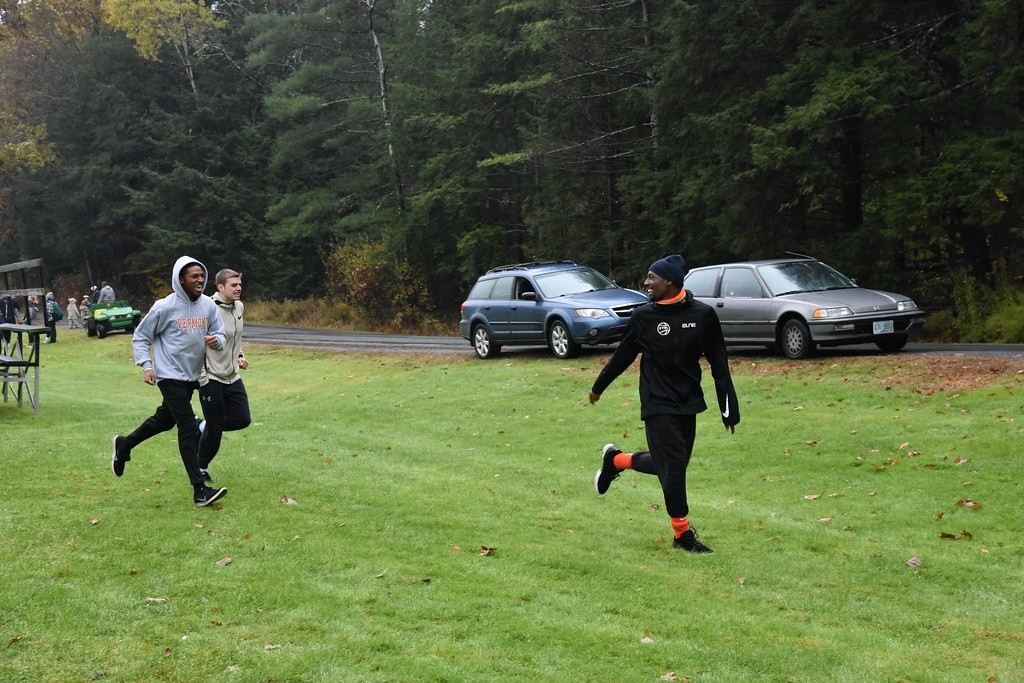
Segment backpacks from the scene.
[48,301,63,320]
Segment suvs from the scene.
[459,261,656,357]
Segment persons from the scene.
[42,292,57,344]
[23,298,38,349]
[111,254,228,508]
[80,281,117,327]
[194,269,251,482]
[0,295,21,348]
[67,298,81,329]
[589,255,741,553]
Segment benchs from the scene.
[0,322,51,416]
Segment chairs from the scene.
[518,280,535,299]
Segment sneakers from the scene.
[112,435,131,477]
[197,454,211,480]
[595,443,626,494]
[194,487,227,507]
[195,414,204,444]
[673,530,714,553]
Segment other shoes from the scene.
[43,337,51,343]
[50,340,56,343]
[26,343,33,348]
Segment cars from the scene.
[83,301,141,338]
[675,258,924,358]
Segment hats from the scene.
[649,255,685,289]
[46,292,54,302]
